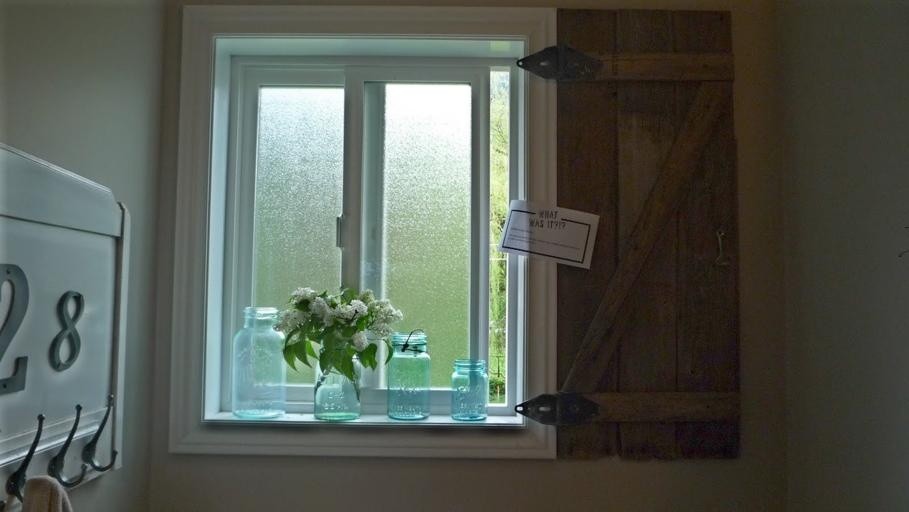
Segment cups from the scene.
[386,331,432,420]
[451,358,490,421]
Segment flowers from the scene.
[273,286,401,384]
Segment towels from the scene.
[21,476,72,512]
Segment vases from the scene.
[315,347,364,421]
[386,334,490,421]
